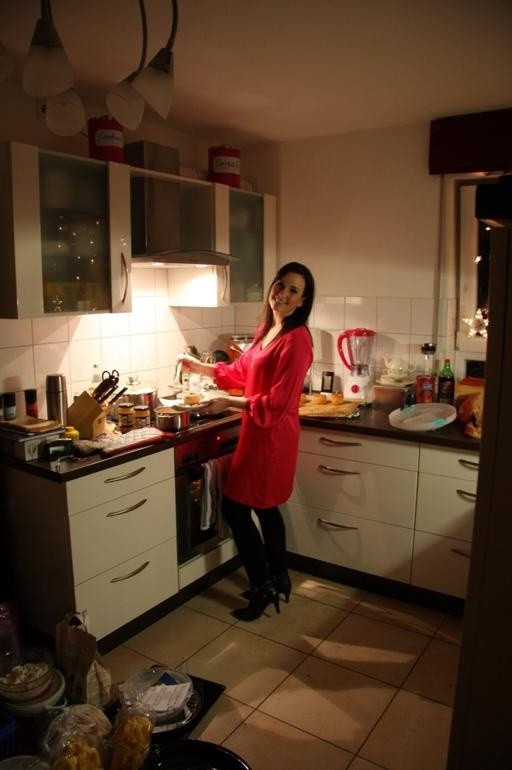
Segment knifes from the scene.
[92,370,128,405]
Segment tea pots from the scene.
[382,353,416,380]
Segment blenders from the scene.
[337,327,375,404]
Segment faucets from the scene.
[231,345,246,355]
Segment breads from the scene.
[299,393,307,403]
[332,393,343,404]
[313,393,327,404]
[184,392,200,405]
[52,716,151,770]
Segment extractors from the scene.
[133,176,242,266]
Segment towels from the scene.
[201,454,234,538]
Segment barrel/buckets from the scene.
[207,144,241,189]
[88,115,124,163]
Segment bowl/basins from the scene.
[5,668,66,717]
[0,662,53,702]
[144,739,251,770]
[0,755,49,770]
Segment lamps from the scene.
[23,0,178,135]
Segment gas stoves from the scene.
[151,403,244,449]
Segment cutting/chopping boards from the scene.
[296,391,362,418]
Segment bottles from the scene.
[60,426,79,443]
[420,343,436,374]
[24,388,38,417]
[415,374,433,404]
[45,374,68,426]
[0,391,17,420]
[437,357,454,406]
[321,370,334,393]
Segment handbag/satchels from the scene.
[55,610,111,707]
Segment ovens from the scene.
[173,424,250,567]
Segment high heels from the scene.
[244,573,292,603]
[234,583,280,621]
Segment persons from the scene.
[179,262,314,621]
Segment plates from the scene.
[164,390,230,415]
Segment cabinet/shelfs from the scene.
[65,447,179,657]
[411,444,480,615]
[279,427,420,600]
[168,182,277,308]
[10,141,131,318]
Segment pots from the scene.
[154,406,191,430]
[112,380,160,421]
